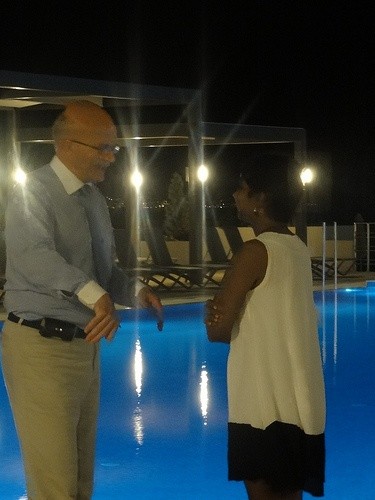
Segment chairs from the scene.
[120,254,357,291]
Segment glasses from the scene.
[71,140,120,155]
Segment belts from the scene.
[7,312,87,340]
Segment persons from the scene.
[205,152,327,500]
[0,100,164,500]
